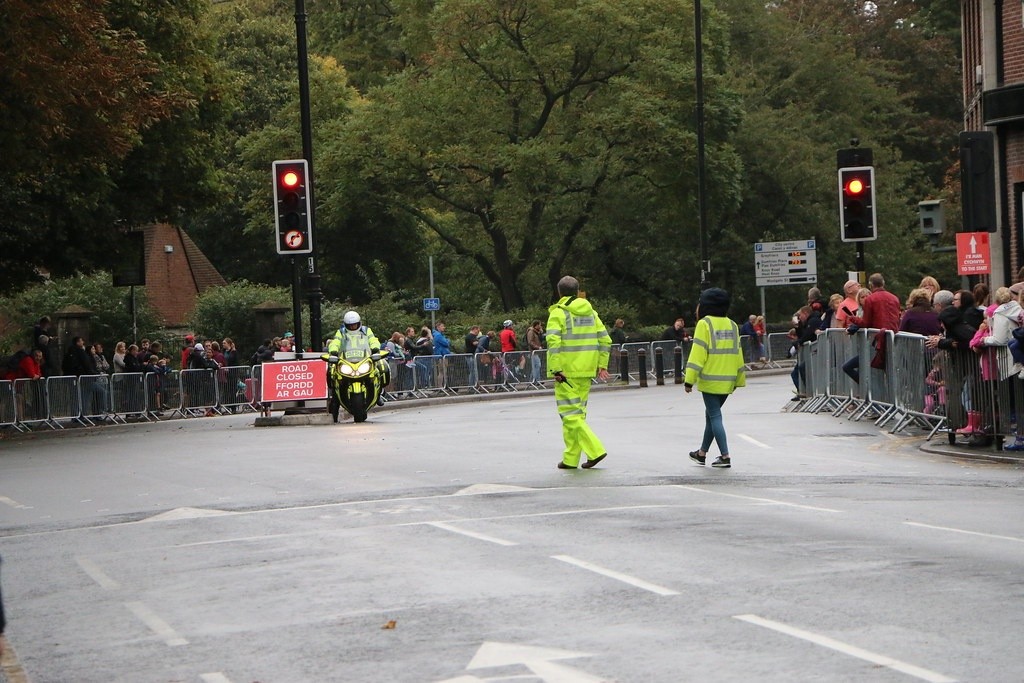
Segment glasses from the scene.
[953,298,960,304]
[922,284,935,289]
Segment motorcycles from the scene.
[320,330,392,422]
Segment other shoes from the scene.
[376,398,384,406]
[558,462,578,469]
[70,403,248,424]
[791,393,1023,453]
[581,453,606,468]
[330,412,339,423]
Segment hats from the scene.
[503,320,512,327]
[284,332,293,339]
[184,335,194,342]
[272,337,282,343]
[698,287,732,318]
[194,343,205,351]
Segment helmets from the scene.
[343,310,363,335]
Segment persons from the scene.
[324,322,451,401]
[525,320,544,386]
[0,315,297,428]
[740,314,757,370]
[786,272,1023,451]
[750,316,766,366]
[684,287,747,468]
[611,319,629,379]
[475,330,496,384]
[465,326,480,390]
[328,308,384,406]
[500,319,518,383]
[660,319,692,375]
[546,276,612,469]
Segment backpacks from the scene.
[523,329,536,348]
[8,350,29,371]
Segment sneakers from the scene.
[711,455,731,467]
[689,449,706,465]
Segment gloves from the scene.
[371,348,379,355]
[331,351,338,357]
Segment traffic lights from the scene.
[839,166,878,242]
[272,159,313,253]
